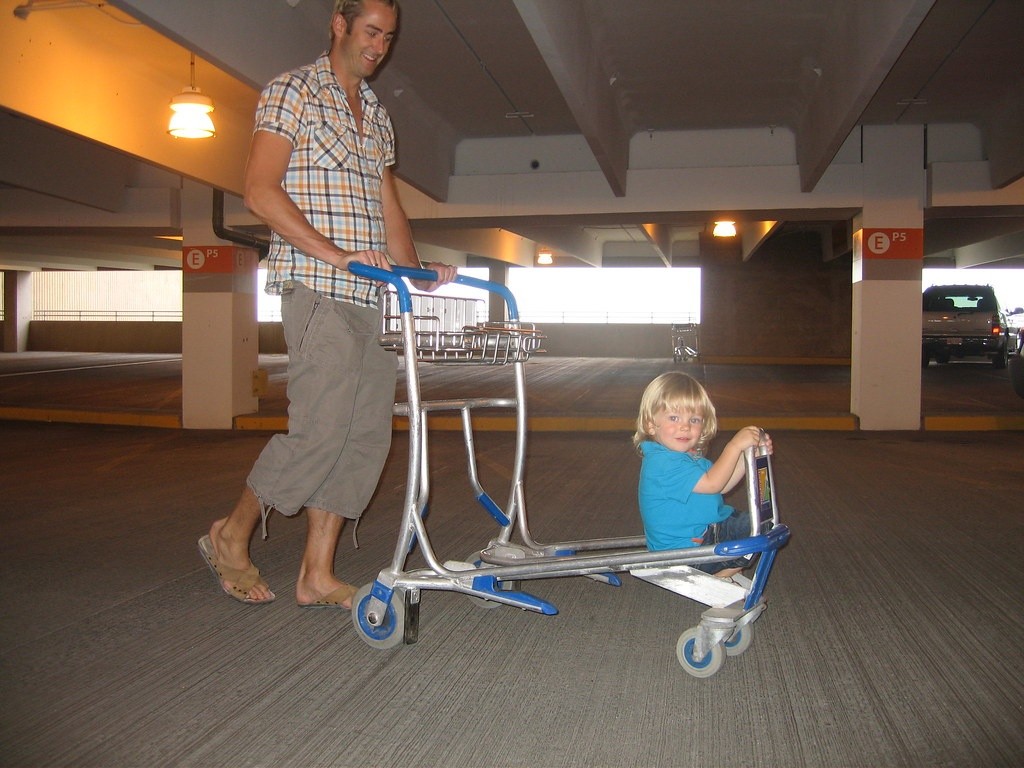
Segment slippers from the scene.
[196,535,276,604]
[297,582,360,611]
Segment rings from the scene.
[372,263,378,266]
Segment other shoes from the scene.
[713,574,742,587]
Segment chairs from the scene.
[977,299,984,308]
[931,299,954,309]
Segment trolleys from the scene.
[348,259,792,678]
[672,322,701,363]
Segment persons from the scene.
[197,0,457,610]
[633,373,774,585]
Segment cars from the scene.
[921,285,1024,371]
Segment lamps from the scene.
[167,51,218,139]
[712,221,737,237]
[535,248,557,265]
